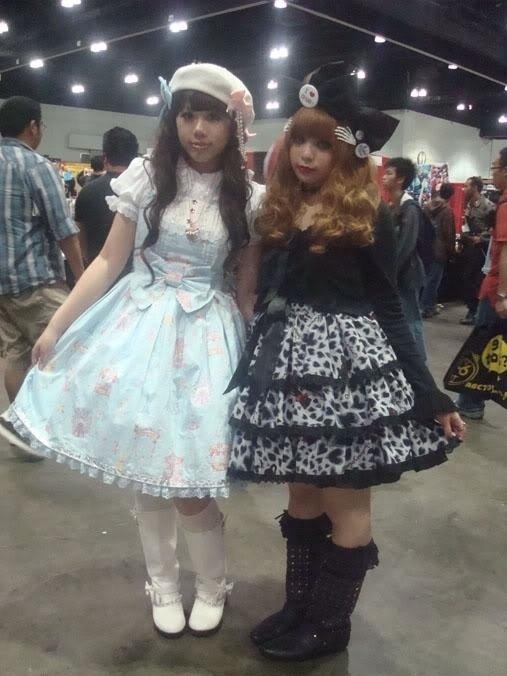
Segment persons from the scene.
[422,182,456,318]
[221,57,468,665]
[1,59,278,641]
[73,126,140,293]
[1,94,87,407]
[78,155,106,187]
[457,174,497,326]
[380,156,428,368]
[450,145,507,420]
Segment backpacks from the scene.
[398,199,436,269]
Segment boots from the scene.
[251,509,332,645]
[177,512,234,636]
[130,502,187,638]
[258,535,379,663]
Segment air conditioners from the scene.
[67,134,103,151]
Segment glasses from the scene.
[41,120,48,129]
[491,164,506,171]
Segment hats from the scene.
[157,62,258,163]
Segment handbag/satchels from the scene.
[444,309,507,410]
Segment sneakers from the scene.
[9,442,43,462]
[453,393,486,420]
[422,302,479,325]
[0,417,46,456]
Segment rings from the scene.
[455,422,466,432]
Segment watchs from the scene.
[496,292,507,302]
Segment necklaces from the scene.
[300,181,322,194]
[184,166,220,241]
